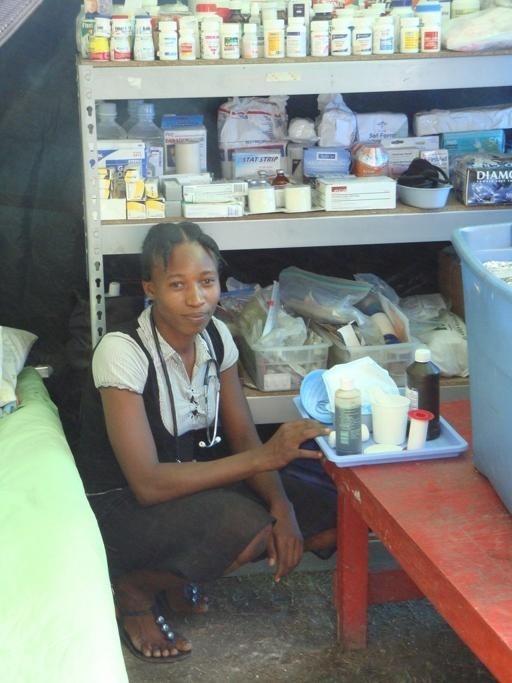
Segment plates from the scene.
[293,391,468,468]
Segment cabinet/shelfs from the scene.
[76,53,512,428]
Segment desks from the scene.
[315,393,512,683]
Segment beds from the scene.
[2,366,128,681]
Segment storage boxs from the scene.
[454,222,512,518]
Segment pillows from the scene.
[1,323,38,412]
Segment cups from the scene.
[371,394,410,445]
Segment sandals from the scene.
[190,586,213,615]
[111,584,193,664]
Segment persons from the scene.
[76,222,340,665]
